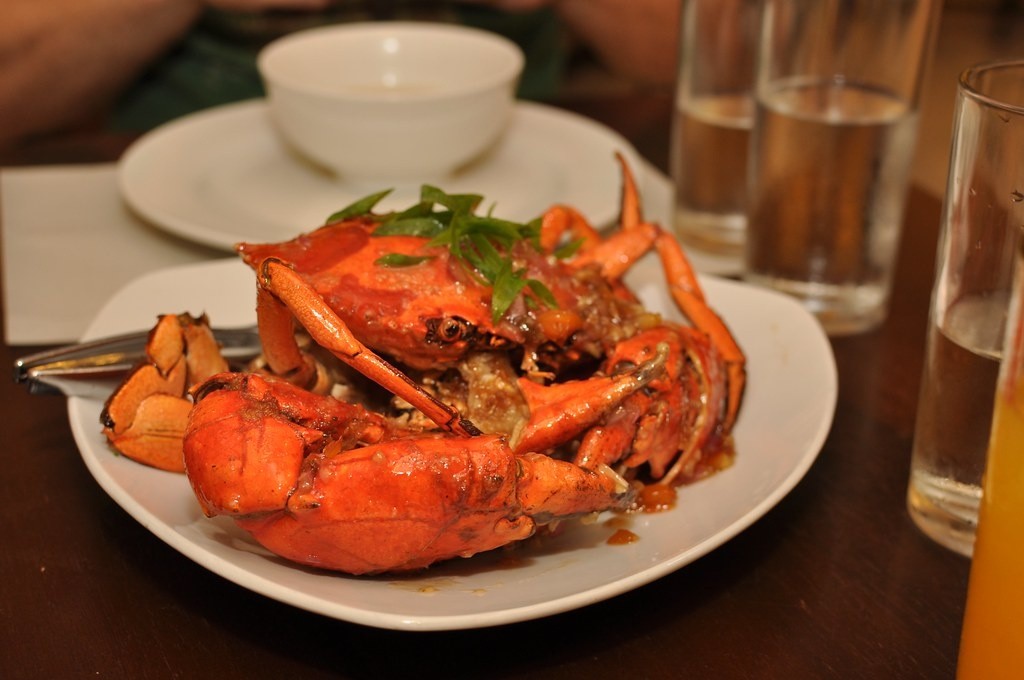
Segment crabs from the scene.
[100,159,746,576]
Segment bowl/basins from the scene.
[257,20,527,177]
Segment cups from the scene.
[905,62,1024,560]
[745,1,942,338]
[667,0,760,257]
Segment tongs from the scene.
[13,326,259,392]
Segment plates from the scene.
[64,258,840,633]
[115,92,639,252]
